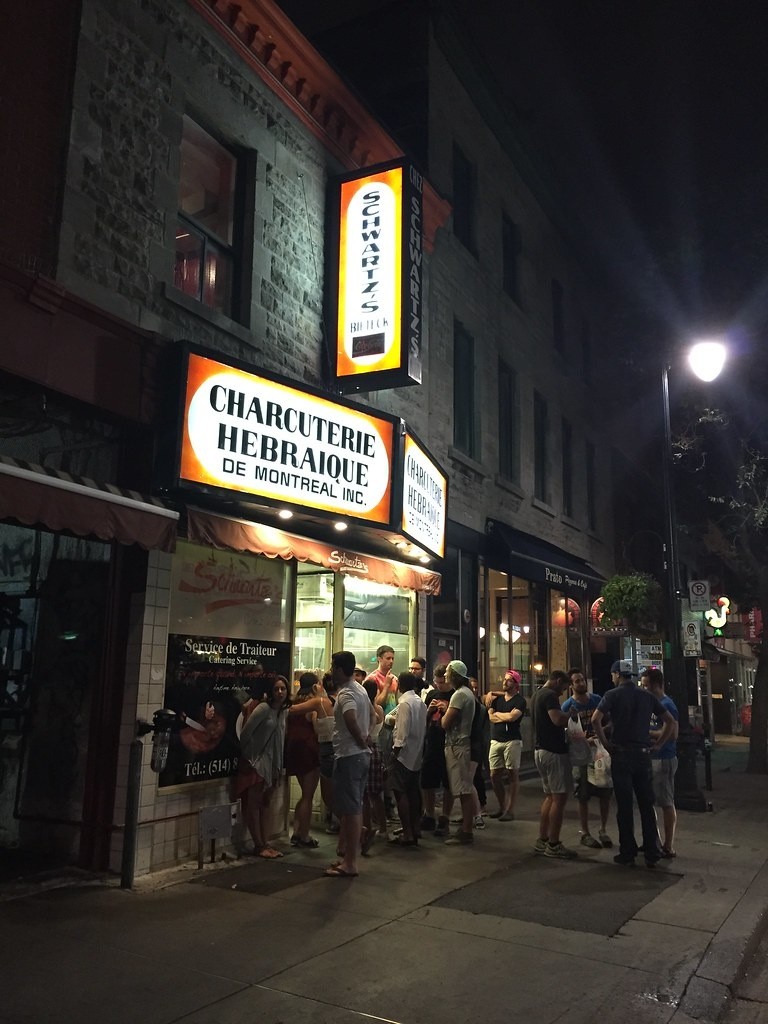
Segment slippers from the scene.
[321,860,360,877]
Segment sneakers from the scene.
[534,839,547,851]
[544,842,578,859]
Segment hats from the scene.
[296,672,319,695]
[449,660,470,680]
[355,667,366,681]
[611,660,639,677]
[506,670,521,691]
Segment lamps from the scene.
[704,597,730,639]
[135,708,176,772]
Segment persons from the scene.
[561,669,613,849]
[590,660,675,867]
[364,645,400,827]
[439,660,476,844]
[390,673,427,848]
[488,670,526,821]
[638,669,679,858]
[322,651,376,876]
[233,644,505,858]
[529,670,577,859]
[289,673,320,848]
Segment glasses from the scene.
[409,666,424,672]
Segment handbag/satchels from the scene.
[238,756,253,775]
[563,714,593,765]
[588,739,613,788]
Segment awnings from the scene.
[0,456,179,554]
[485,516,605,599]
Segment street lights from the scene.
[661,340,728,813]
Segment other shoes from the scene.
[646,849,662,868]
[499,812,515,821]
[599,829,613,849]
[638,844,644,850]
[614,854,635,866]
[324,808,489,856]
[661,846,676,857]
[255,845,284,858]
[489,813,502,818]
[580,832,602,850]
[291,835,318,849]
